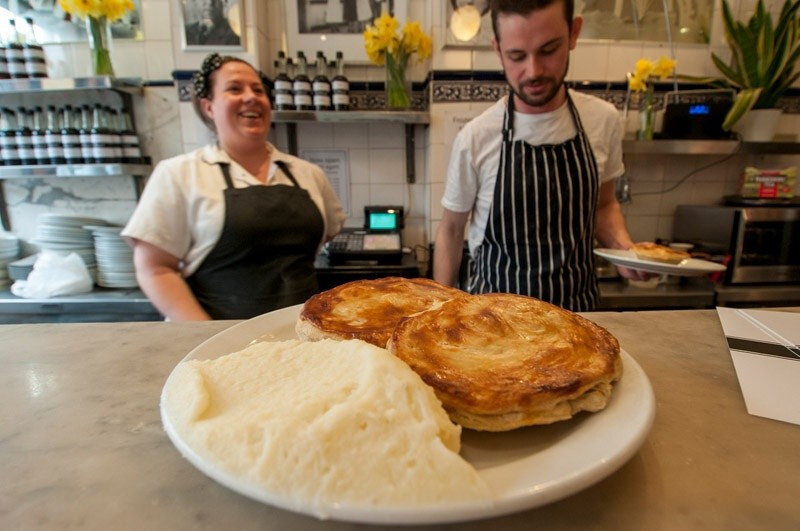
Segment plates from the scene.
[1,214,137,289]
[158,303,656,525]
[591,246,728,277]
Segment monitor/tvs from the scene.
[364,205,404,231]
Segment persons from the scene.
[119,51,348,322]
[183,1,240,46]
[426,0,658,314]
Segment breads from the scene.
[630,243,690,264]
[292,277,622,434]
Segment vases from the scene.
[382,44,411,111]
[84,14,114,75]
[639,87,656,142]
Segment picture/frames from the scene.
[284,0,412,64]
[177,0,245,52]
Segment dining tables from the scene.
[3,317,800,529]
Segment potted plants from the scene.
[662,0,800,144]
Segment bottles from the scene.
[270,50,350,110]
[0,102,144,165]
[23,17,47,77]
[0,39,9,79]
[6,18,27,78]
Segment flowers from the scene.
[56,0,135,76]
[628,53,677,144]
[363,11,432,112]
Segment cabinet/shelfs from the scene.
[0,75,156,194]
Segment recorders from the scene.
[645,0,736,140]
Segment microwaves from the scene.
[673,203,799,285]
